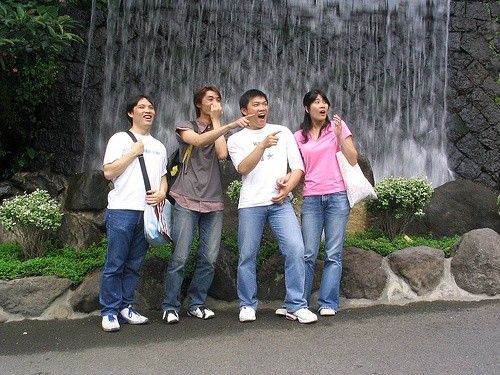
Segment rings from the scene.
[338,120,341,124]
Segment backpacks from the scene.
[166,120,198,205]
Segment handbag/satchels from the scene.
[356,152,375,187]
[143,199,173,249]
[335,151,378,208]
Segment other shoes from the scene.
[320,308,335,316]
[275,308,287,316]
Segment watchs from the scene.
[337,142,345,147]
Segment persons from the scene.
[97,91,170,333]
[159,84,256,326]
[226,88,319,328]
[274,87,359,317]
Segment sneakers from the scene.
[117,304,149,325]
[187,305,215,320]
[286,307,318,324]
[162,310,179,324]
[239,306,256,321]
[102,315,120,332]
